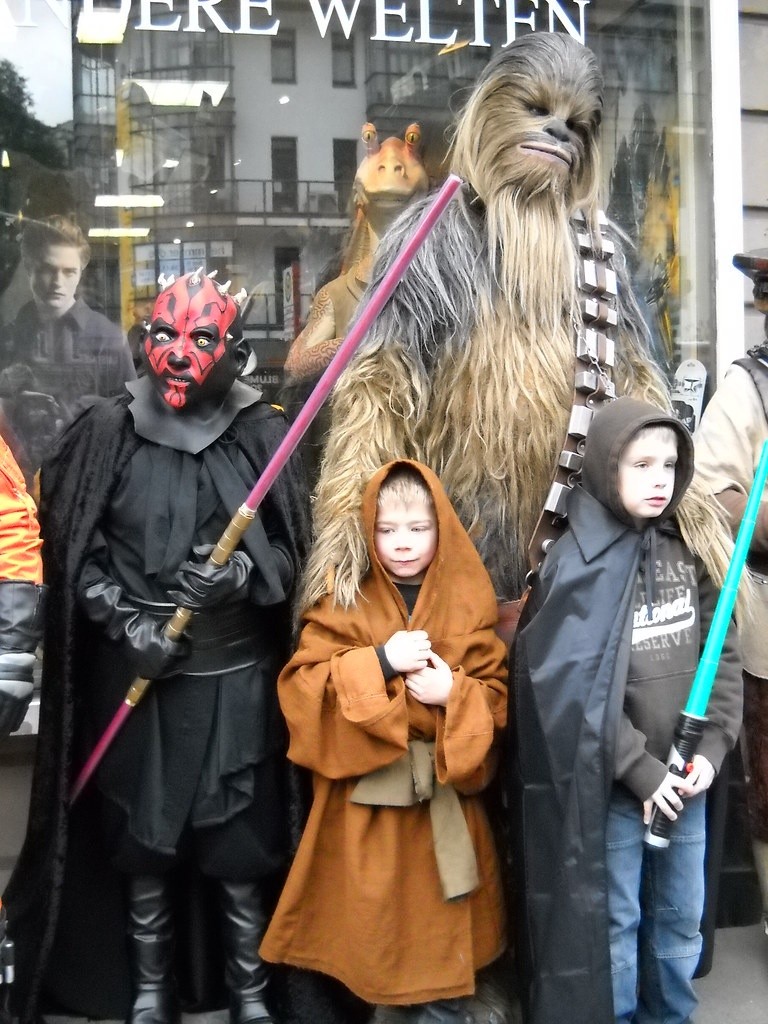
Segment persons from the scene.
[0,214,138,475]
[0,436,44,743]
[523,397,745,1024]
[691,249,768,936]
[278,459,509,1024]
[40,269,305,1024]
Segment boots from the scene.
[129,871,181,1024]
[222,880,274,1024]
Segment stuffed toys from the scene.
[290,31,758,646]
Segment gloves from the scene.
[82,582,192,680]
[165,543,254,609]
[0,581,51,740]
